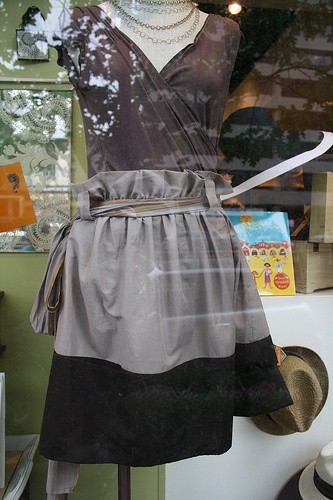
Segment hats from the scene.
[251,347,329,434]
[298,444,333,500]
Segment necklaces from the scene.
[109,0,200,43]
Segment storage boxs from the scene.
[290,172,333,294]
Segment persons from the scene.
[29,0,294,500]
